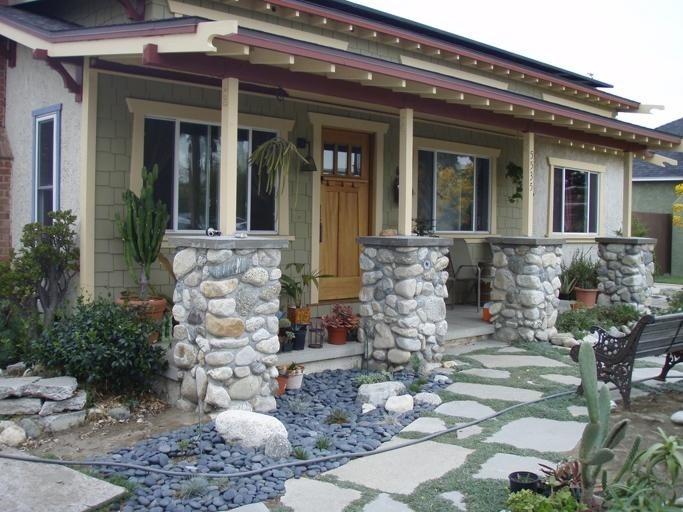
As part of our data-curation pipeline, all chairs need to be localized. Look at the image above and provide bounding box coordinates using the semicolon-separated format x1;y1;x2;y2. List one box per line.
446;237;481;312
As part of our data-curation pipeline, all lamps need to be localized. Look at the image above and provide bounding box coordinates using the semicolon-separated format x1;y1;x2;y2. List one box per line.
292;133;319;176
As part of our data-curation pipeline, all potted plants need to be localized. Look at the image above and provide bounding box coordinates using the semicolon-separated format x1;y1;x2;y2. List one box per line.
110;160;171;343
574;262;603;305
508;470;582;503
556;272;573;301
275;255;325;322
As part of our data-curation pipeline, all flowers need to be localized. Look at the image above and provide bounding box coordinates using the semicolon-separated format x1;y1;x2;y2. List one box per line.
320;304;356;329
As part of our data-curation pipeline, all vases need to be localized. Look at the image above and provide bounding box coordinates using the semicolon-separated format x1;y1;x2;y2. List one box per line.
276;375;288;395
279;326;307;352
329;324;348;346
281;365;305;390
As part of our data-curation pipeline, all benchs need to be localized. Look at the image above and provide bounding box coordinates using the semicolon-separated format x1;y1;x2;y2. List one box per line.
568;309;682;410
